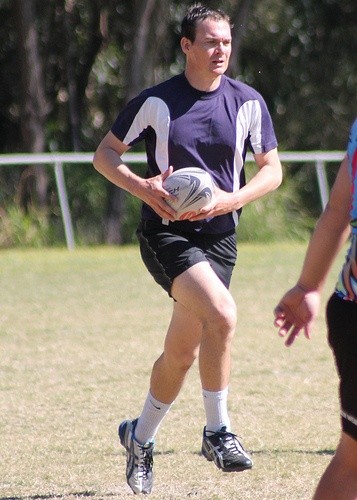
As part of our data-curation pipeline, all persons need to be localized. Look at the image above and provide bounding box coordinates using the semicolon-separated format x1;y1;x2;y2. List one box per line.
273;114;357;500
93;5;285;495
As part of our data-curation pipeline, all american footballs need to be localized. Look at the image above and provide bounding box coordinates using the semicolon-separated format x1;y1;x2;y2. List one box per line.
163;167;217;221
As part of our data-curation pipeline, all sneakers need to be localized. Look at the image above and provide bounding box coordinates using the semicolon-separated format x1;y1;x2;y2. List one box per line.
118;418;155;495
202;426;254;472
